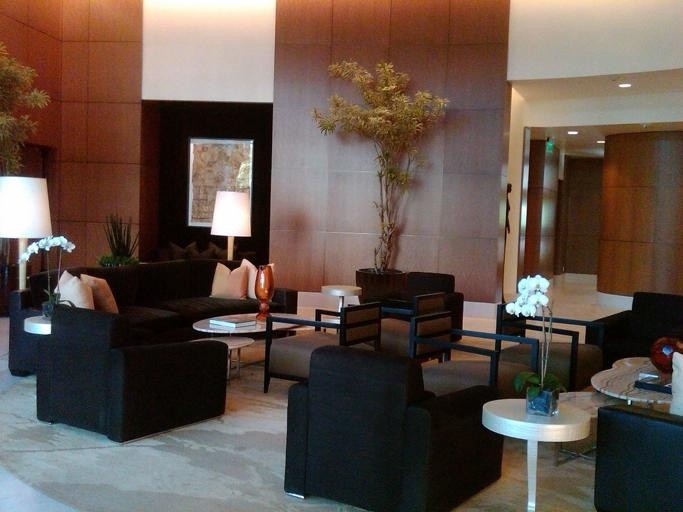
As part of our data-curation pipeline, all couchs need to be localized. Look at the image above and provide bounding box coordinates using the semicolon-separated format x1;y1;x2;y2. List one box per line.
584;284;683;511
280;342;507;511
396;273;464;343
6;259;298;381
33;305;229;444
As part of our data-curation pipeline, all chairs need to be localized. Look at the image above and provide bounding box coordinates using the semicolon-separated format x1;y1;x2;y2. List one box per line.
406;300;600;465
260;293;454;395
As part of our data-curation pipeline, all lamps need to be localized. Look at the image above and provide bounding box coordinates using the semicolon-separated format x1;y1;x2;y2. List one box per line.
0;171;59;310
207;186;252;260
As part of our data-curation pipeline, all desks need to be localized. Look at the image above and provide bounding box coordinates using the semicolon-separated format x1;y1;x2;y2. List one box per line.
192;310;308;378
482;354;676;512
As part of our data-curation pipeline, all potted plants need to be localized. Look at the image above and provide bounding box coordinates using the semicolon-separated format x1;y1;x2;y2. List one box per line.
507;273;562;418
312;58;448;300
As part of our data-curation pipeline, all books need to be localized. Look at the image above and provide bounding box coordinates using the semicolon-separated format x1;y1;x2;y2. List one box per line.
208;315;256;329
634;375;673;395
638;369;659;378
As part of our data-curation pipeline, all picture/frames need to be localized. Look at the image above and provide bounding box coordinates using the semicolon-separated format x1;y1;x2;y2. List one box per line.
185;135;254;228
